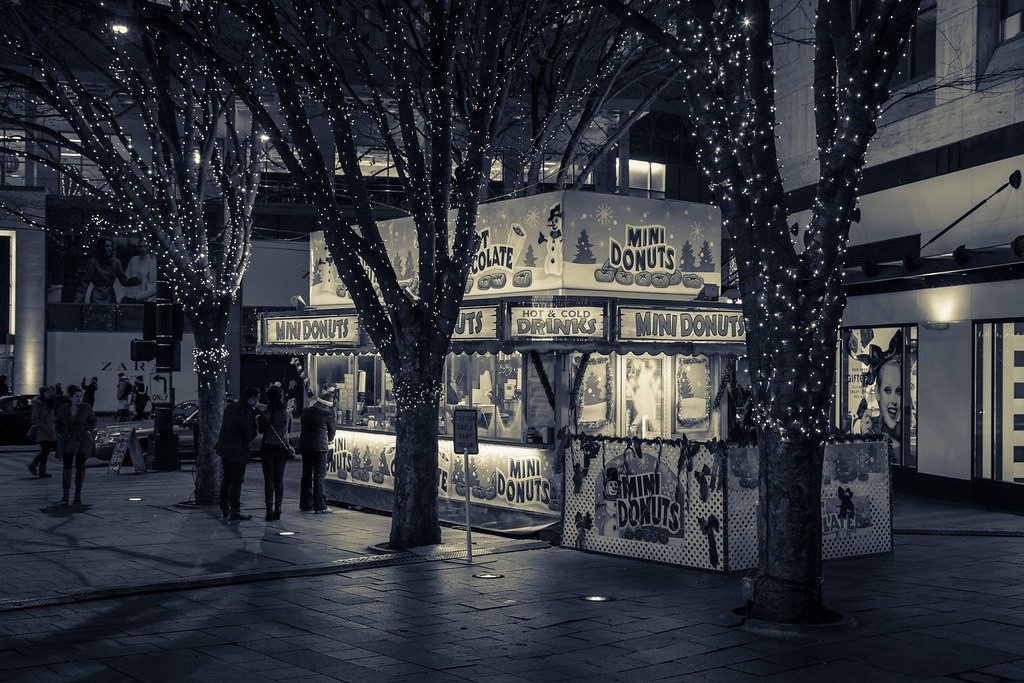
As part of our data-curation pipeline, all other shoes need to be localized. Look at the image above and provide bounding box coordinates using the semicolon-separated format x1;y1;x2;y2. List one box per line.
230;511;253;520
315;507;332;514
265;511;273;522
273;510;281;520
223;506;231;518
301;505;314;512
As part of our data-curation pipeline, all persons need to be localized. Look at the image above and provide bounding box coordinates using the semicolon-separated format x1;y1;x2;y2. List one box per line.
0;375;8;397
54;384;64;395
114;238;157;304
29;386;56;478
855;359;901;463
215;386;260;520
72;238;140;304
81;377;98;407
55;386;97;508
299;393;335;513
257;386;288;521
129;376;149;420
118;373;132;422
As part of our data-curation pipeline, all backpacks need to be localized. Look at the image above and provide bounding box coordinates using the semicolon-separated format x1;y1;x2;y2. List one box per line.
122;379;132;394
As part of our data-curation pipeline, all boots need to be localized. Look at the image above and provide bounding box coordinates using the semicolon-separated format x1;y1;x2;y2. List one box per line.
38;465;52;478
60;471;71;504
73;470;84;507
27;458;40;476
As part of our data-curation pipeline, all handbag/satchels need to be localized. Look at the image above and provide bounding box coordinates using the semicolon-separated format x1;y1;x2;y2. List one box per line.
286;446;295;459
27;424;38;441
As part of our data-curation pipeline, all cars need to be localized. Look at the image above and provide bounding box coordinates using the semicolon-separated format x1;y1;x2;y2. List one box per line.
88;399;302;470
0;392;98;443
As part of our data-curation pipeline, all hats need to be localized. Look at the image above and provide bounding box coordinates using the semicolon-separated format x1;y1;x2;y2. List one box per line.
136;376;143;382
317;393;333;406
40;386;50;395
92;377;98;384
